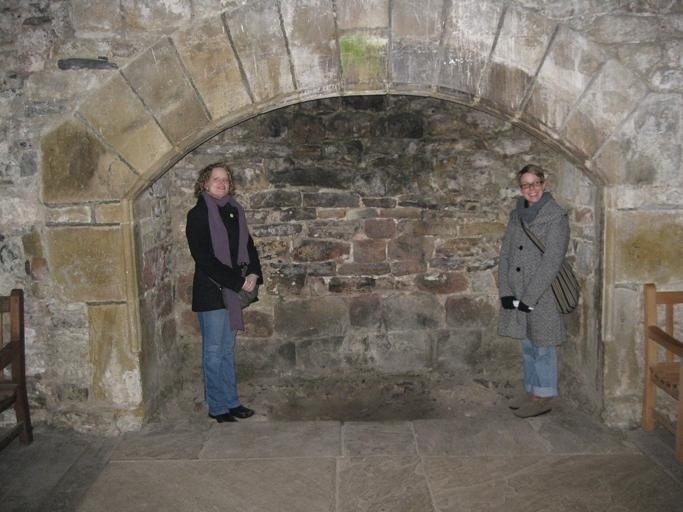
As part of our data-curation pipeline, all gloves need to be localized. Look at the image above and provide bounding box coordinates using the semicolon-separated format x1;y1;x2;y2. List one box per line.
517;300;532;313
501;296;517;309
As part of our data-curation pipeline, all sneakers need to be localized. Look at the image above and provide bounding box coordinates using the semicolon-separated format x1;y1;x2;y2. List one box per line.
208;405;254;423
508;393;552;418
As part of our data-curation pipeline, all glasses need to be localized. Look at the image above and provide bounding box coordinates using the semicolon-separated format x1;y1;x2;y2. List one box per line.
520;181;543;188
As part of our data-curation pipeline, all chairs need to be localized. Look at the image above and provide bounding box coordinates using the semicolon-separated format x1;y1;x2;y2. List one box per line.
0;288;33;444
643;283;682;459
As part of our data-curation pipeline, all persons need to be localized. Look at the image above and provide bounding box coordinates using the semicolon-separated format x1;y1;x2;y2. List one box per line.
498;164;571;417
186;162;263;422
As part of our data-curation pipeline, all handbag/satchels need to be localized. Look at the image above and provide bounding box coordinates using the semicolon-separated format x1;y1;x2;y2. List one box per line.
551;262;580;314
222;266;258;311
